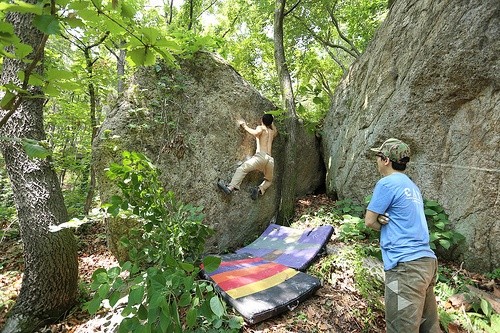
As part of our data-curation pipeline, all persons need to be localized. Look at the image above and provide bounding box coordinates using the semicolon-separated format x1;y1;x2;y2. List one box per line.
365;138;444;333
217;113;278;201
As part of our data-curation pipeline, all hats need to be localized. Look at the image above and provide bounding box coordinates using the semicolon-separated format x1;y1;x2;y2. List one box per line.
370;138;412;164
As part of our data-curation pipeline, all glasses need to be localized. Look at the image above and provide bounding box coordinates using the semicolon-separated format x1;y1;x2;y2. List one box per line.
375;154;385;161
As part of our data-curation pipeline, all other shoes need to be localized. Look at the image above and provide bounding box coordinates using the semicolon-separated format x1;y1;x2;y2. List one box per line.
251;187;258;201
217;183;232;195
